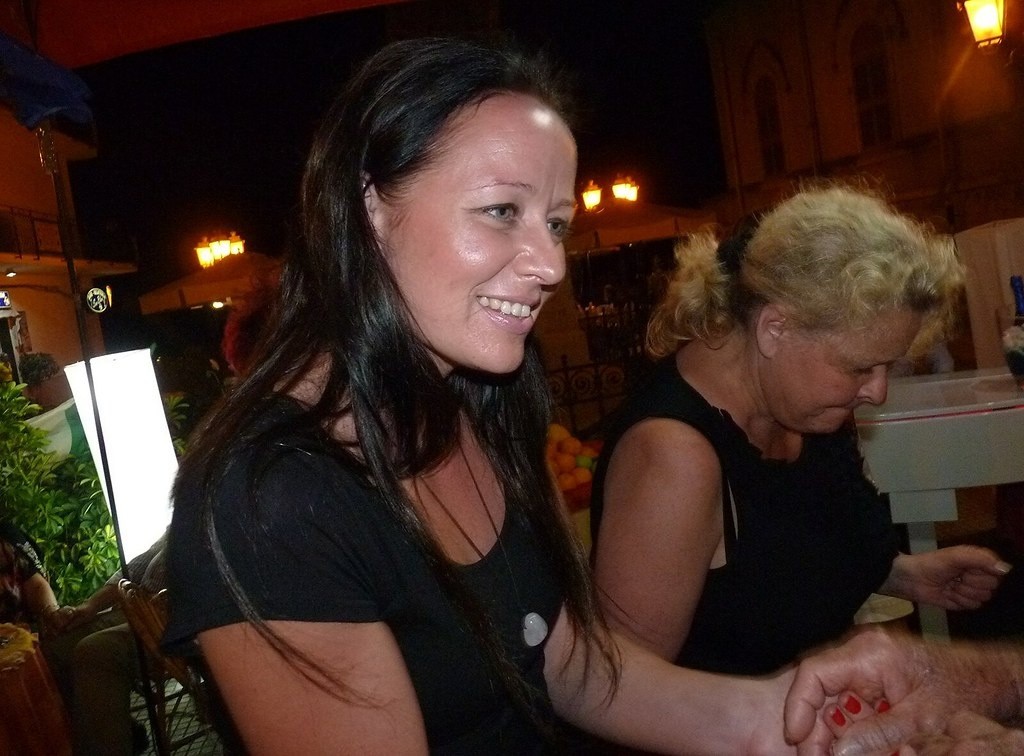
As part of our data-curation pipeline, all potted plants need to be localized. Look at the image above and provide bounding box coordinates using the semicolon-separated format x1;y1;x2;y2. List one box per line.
19;352;66;410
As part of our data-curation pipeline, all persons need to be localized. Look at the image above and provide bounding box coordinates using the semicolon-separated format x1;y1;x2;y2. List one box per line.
784;627;1024;756
164;38;590;756
590;187;1014;675
42;533;167;756
0;522;75;631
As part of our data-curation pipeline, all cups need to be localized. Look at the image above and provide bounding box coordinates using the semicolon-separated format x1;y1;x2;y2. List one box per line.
996;299;1024;391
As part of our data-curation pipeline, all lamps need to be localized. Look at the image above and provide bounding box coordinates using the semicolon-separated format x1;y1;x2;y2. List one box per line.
581;173;640;214
961;0;1007;49
193;228;250;275
63;345;179;564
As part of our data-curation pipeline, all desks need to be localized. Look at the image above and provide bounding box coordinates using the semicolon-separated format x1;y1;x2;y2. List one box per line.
0;621;76;756
853;366;1024;644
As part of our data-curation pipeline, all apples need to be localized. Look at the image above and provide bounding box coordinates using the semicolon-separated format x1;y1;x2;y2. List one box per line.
545;423;600;492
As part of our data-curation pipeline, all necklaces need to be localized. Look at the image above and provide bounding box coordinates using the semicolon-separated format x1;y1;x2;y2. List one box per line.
422;445;547;646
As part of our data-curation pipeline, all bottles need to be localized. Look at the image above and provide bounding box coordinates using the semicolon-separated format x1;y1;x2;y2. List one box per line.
1010;276;1024;326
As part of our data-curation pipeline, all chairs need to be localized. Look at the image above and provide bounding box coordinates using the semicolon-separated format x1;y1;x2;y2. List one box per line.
134;588;215;755
116;578;196;694
